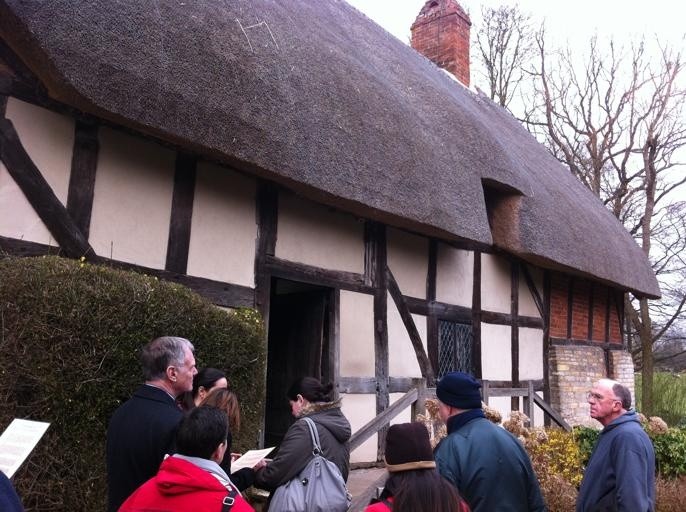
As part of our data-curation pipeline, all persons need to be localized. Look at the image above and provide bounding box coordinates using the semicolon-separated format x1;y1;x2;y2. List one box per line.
575;379;655;511
106;336;257;512
254;377;351;512
432;372;549;512
362;422;471;512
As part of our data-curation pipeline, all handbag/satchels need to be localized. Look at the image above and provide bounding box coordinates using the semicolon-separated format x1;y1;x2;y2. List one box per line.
266;415;353;512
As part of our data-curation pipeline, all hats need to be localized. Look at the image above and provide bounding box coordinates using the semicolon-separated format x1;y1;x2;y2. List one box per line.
382;421;438;472
435;370;484;410
